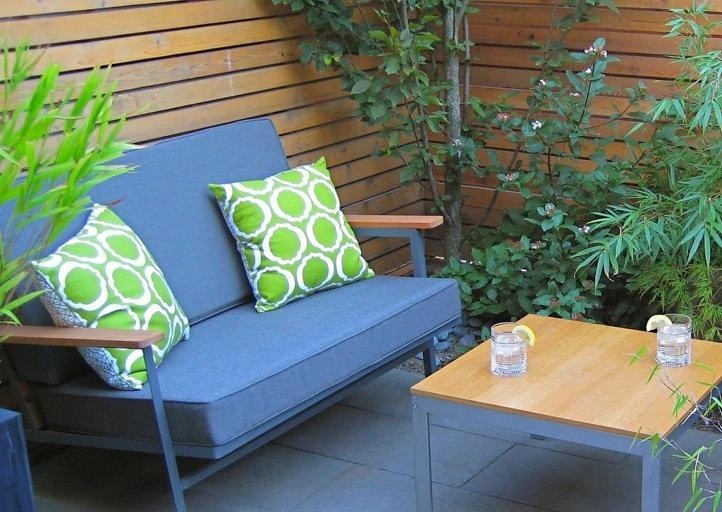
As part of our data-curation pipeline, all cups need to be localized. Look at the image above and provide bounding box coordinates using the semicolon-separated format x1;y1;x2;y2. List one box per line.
490;322;527;377
657;313;692;368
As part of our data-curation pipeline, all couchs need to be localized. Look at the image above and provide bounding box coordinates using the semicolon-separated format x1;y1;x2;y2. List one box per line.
0;118;463;512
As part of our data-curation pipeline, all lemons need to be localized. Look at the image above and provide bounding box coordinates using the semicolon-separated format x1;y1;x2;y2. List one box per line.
646;314;672;332
512;324;535;348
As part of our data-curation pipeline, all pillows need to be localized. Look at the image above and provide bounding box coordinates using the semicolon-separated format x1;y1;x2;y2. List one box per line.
27;203;191;390
208;156;375;314
